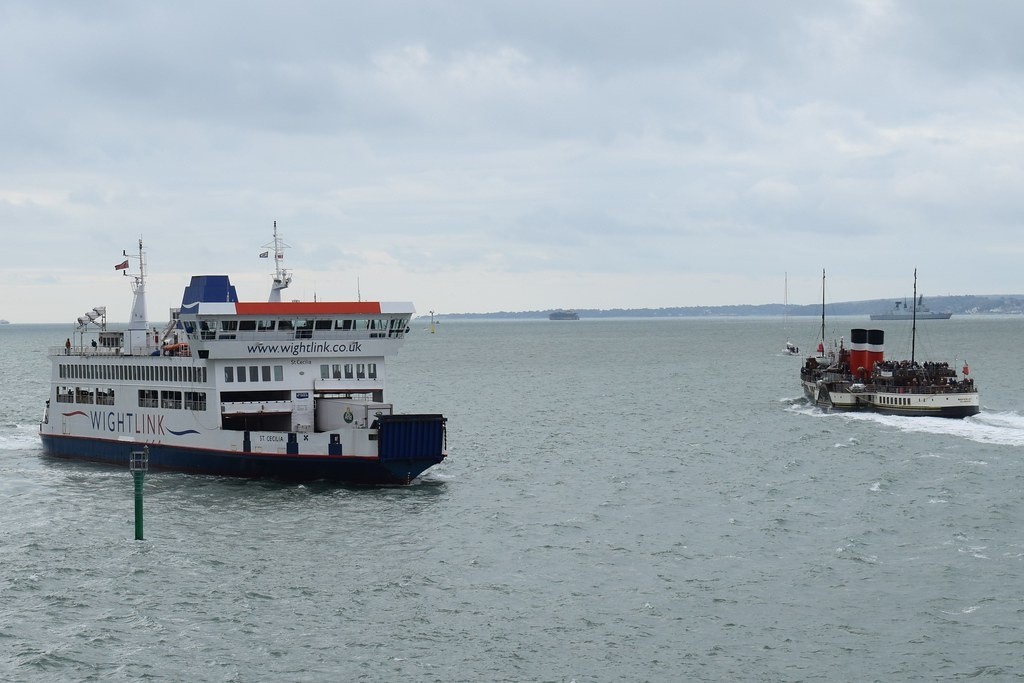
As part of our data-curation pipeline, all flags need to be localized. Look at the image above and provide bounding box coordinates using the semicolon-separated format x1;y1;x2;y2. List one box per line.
115;260;129;271
260;251;268;259
962;363;969;375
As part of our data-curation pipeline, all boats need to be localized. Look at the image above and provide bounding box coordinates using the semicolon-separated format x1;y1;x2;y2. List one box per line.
799;268;871;413
435;321;440;327
423;328;429;331
37;234;448;489
430;311;436;334
845;267;982;419
170;221;416;365
870;294;952;320
549;311;580;320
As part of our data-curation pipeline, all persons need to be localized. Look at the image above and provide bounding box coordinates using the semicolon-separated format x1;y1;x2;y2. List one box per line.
872;357;949;370
801;361;828;381
871;374;975;394
65;338;71;356
67;389;73;398
92;339;97;348
792;346;799;353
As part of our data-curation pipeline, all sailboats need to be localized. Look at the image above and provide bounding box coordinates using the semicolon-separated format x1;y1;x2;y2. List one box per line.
781;272;803;357
